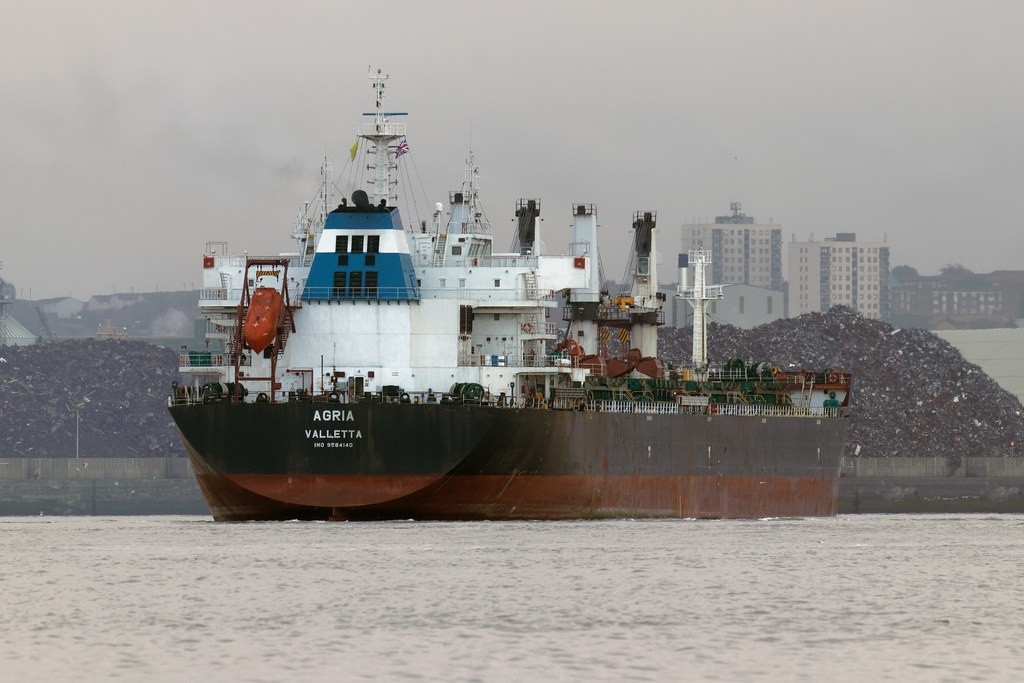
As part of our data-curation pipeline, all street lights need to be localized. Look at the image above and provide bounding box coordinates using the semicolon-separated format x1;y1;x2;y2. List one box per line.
76;403;84;460
1011;442;1014;478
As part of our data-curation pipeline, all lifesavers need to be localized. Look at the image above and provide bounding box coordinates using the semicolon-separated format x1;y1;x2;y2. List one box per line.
523;323;531;332
828;374;837;383
771;367;785;379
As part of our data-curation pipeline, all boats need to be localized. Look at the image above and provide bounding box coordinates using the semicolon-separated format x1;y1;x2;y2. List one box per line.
243;287;285;355
167;66;853;523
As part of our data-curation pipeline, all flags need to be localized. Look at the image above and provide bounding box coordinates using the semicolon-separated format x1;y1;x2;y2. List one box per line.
396;139;410;159
349;140;359;160
